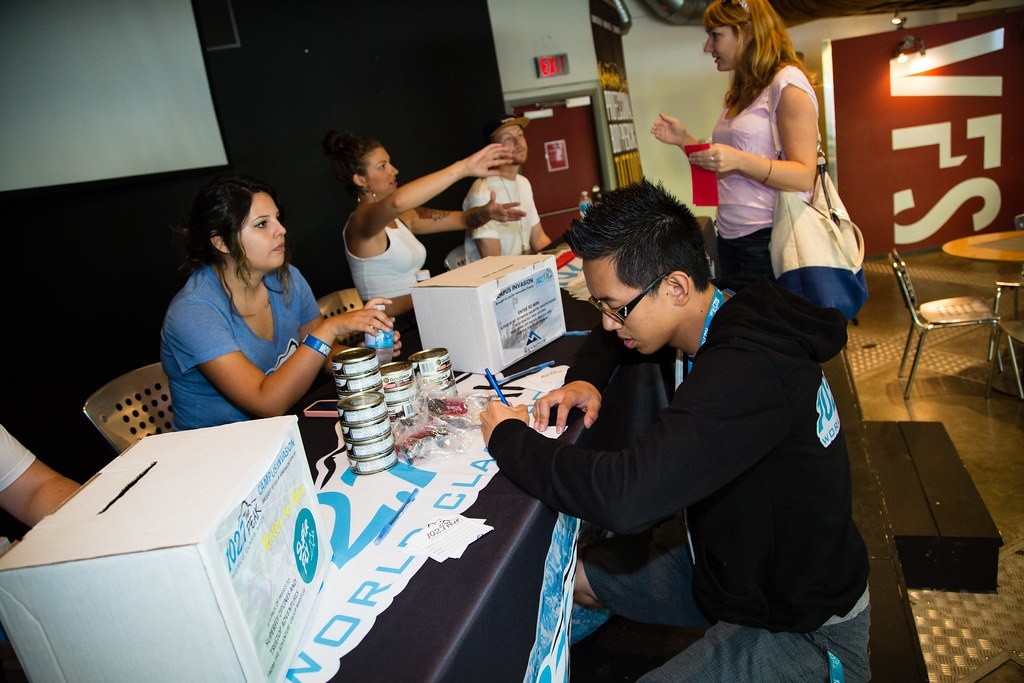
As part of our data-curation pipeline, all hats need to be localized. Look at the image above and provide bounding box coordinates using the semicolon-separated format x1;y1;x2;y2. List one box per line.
482;113;530;144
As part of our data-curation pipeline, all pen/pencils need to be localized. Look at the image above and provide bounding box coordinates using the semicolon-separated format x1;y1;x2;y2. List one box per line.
374;487;419;545
485;368;509;407
563;331;589;336
497;360;556;386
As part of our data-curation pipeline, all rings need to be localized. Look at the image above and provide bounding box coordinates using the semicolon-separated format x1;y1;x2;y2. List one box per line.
710;155;714;162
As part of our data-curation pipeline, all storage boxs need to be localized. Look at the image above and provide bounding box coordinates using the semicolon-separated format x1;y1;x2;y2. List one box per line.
409;253;567;375
0;414;333;683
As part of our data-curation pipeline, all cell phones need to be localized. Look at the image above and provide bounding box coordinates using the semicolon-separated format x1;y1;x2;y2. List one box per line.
303;400;340;418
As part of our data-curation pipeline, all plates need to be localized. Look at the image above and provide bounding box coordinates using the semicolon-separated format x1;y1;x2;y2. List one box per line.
364;304;394;367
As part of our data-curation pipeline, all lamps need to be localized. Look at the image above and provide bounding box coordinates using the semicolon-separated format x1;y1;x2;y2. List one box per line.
891;5;902;24
892;36;927;64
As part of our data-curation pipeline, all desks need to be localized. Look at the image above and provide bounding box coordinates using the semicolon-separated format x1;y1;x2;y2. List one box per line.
283;216;717;683
942;231;1024;361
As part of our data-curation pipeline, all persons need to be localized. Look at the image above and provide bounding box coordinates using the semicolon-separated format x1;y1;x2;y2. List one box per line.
0;424;82;528
478;177;874;683
158;175;402;432
650;0;820;310
462;113;552;264
321;128;526;306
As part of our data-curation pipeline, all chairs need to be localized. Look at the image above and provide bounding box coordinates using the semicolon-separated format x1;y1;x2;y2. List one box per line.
83;363;175;456
986;280;1024;401
888;247;1004;399
444;244;466;270
316;287;365;346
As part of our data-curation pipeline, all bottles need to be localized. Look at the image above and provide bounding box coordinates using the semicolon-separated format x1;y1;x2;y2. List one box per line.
416;269;431;283
591;185;602;205
580;191;593;223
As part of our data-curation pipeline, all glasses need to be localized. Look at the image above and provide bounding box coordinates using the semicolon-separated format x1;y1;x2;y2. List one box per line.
721;0;742;9
586;272;669;324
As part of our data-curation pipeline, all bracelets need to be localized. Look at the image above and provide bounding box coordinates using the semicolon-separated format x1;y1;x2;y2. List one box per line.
301;333;332;357
761;158;772;183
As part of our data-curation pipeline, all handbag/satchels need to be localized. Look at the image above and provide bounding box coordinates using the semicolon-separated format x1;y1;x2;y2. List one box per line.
769;67;868;320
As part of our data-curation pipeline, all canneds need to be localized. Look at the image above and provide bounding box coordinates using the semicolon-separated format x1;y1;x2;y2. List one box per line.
329;346;459;475
